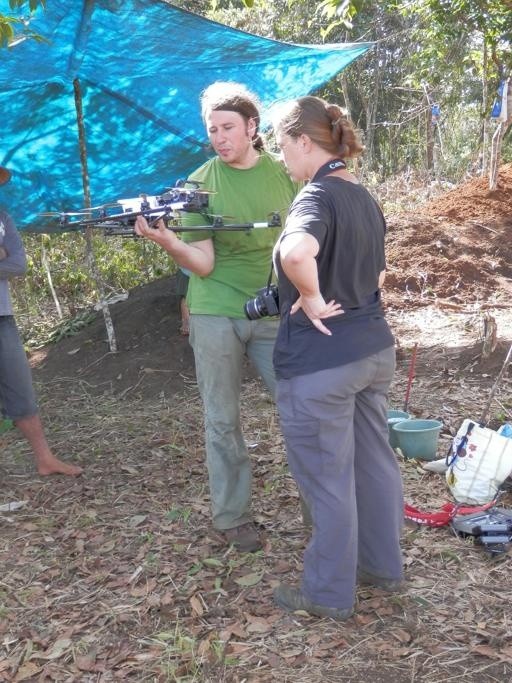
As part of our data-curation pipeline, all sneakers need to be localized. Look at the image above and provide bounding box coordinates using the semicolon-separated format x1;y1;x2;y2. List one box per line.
224;521;266;552
274;578;356;621
355;567;403;591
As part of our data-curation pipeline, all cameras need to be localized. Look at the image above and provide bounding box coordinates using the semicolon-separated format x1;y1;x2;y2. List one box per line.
244;284;279;323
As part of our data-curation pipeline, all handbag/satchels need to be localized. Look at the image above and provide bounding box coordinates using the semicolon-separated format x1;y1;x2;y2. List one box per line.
444;418;511;508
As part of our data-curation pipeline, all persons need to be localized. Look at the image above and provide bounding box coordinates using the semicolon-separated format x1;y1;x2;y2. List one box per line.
266;96;411;619
129;81;303;551
0;198;85;480
176;264;193;337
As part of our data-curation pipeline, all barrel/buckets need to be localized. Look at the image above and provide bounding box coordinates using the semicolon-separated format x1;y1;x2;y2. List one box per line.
392;420;443;461
387;409;411;448
